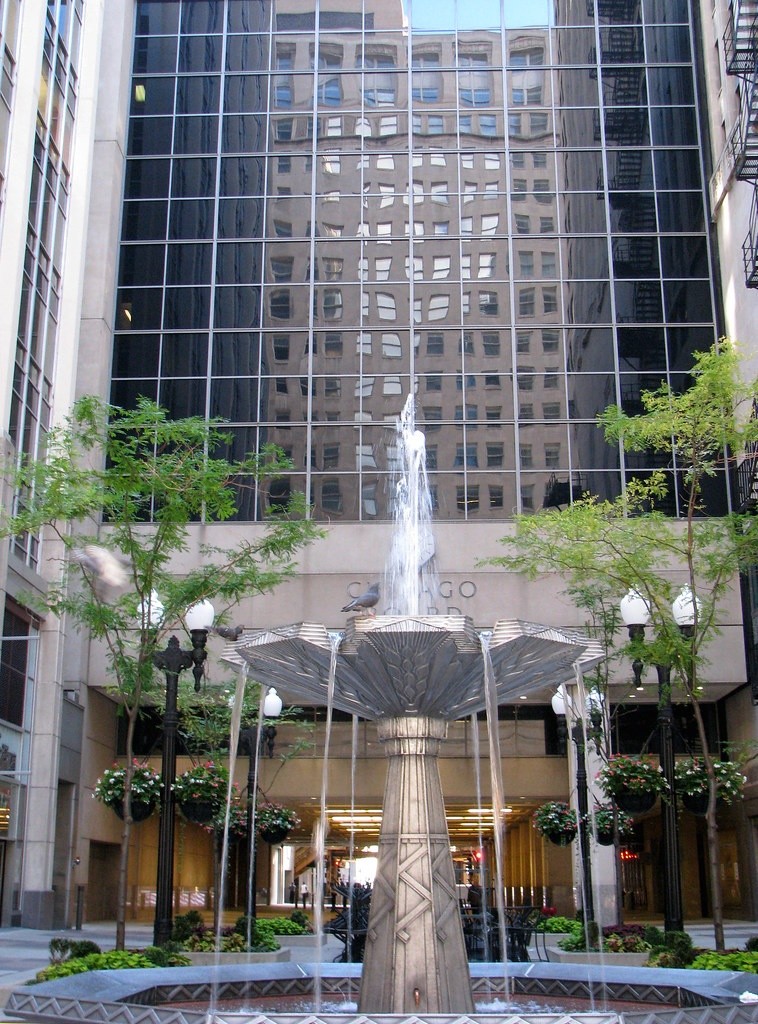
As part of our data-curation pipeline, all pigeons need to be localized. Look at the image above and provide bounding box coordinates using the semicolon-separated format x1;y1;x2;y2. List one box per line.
339;581;382;616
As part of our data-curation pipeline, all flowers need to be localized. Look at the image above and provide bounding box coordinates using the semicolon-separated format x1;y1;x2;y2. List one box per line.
169;760;248;835
254;801;300;836
530;800;583;843
582;802;634;860
558;923;664;953
593;752;671;806
532;906;582;933
674;755;748;806
91;757;164;816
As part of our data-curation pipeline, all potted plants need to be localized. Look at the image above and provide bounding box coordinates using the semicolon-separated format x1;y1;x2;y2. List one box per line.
254;909;328;946
173;910;292;967
0;938;192;1009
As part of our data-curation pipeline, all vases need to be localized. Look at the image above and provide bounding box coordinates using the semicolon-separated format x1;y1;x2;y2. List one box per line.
613;791;659;814
526;933;574;962
682;791;725;814
109;801;155;822
545;946;664;967
180;801;221;824
543;829;576;846
256;818;295;844
590;827;622;846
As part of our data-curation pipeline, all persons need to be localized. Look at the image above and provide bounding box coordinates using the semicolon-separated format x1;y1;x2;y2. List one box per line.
467;878;482;921
300;882;308;909
289;882;296;904
